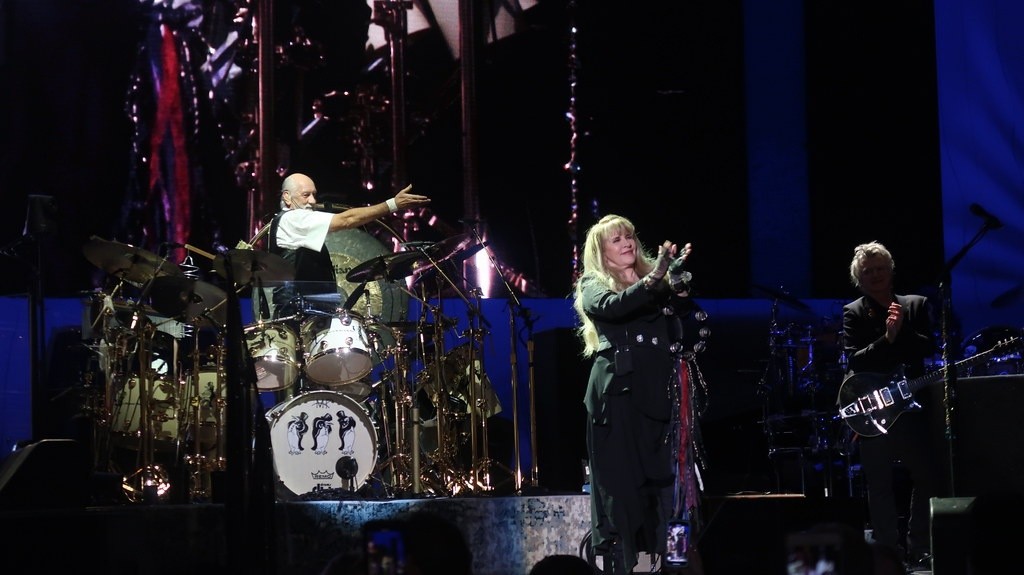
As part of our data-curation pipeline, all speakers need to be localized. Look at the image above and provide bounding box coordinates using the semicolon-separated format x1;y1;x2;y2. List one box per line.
928;497;981;575
0;438;77;505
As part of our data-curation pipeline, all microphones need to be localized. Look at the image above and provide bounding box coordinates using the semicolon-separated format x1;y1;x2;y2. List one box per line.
969;204;1003;228
163;242;185;248
406;241;436;246
457;218;484;223
213;242;231;257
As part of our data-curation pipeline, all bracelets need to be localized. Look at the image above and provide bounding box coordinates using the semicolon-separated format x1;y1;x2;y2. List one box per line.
386;198;399;212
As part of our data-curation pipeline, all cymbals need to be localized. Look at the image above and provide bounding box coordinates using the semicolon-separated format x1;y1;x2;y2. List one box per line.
369;321;432;333
423;232;473;267
141;275;229;326
213;248;297;289
83;240;185;284
344;249;429;283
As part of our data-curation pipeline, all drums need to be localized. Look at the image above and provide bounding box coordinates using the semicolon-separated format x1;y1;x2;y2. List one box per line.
304;374;374;405
109;371;187;453
244;321;299;393
180;364;229;444
268;388;379;499
416;341;504;443
300;311;374;387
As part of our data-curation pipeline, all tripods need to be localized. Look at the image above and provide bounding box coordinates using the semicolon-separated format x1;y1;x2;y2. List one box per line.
83;249;223;505
375;222;533;499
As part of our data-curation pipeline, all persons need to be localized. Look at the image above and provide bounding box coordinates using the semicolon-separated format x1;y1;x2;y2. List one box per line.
320;512;608;575
841;242;939;568
269;173;431;404
573;214;692;575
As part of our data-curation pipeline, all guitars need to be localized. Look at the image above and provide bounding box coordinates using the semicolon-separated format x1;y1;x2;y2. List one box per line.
837;335;1024;438
